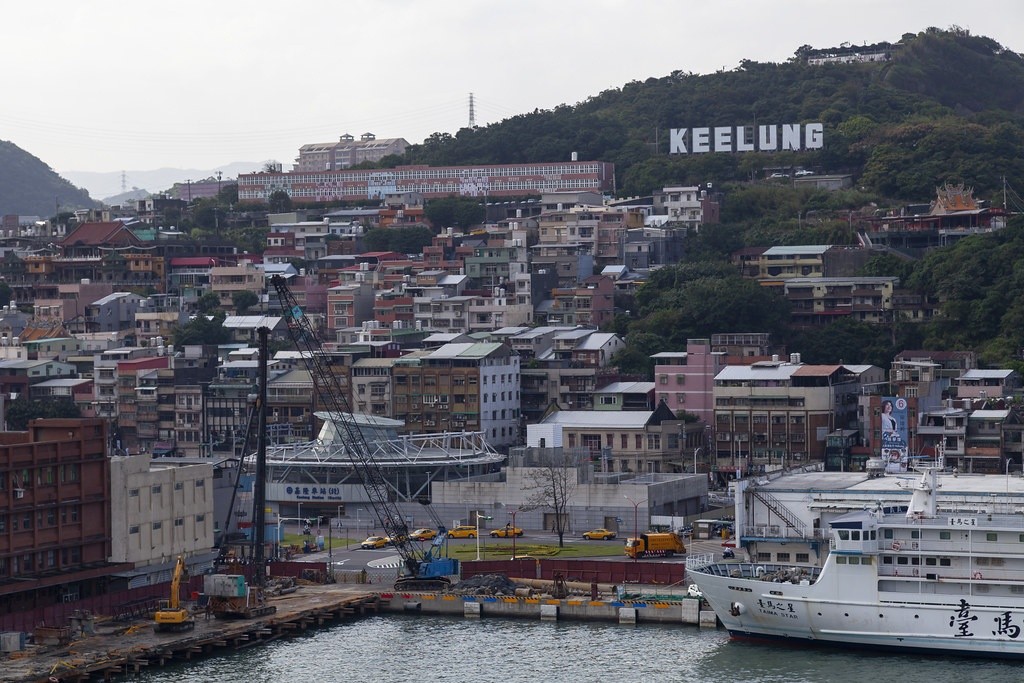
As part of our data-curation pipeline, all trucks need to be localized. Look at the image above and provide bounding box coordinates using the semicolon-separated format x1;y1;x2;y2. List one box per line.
626;533;684;560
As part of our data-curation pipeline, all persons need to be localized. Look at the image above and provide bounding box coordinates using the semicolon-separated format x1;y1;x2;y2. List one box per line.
552;520;556;533
882;401;897;434
504;526;509;538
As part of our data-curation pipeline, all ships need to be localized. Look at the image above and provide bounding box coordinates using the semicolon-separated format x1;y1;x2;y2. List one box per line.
685;434;1024;656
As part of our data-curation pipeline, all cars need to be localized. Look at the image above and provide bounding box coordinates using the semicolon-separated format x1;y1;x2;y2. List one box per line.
493;526;524;537
449;526;478;539
356;536;386;551
584;528;615;538
407;530;436;541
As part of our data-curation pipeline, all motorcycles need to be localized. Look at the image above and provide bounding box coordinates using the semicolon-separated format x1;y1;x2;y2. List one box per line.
722;551;735;557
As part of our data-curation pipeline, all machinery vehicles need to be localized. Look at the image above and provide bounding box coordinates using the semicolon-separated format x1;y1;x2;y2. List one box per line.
271;272;457;593
154;552;196;629
204;327;276;617
